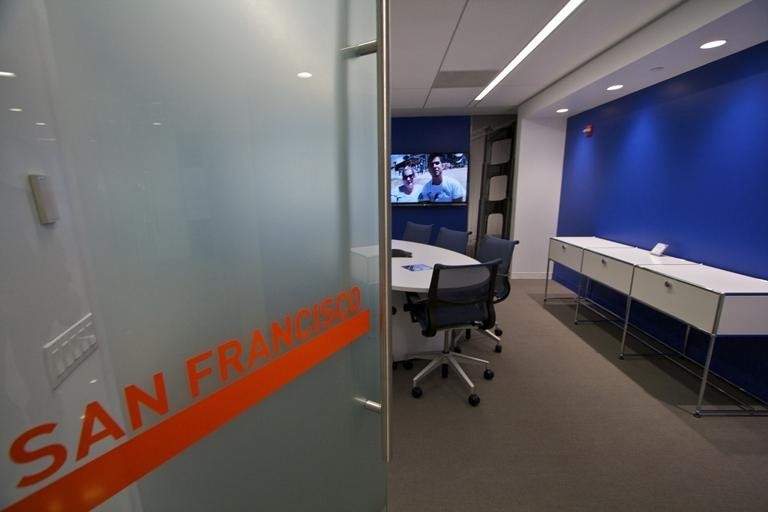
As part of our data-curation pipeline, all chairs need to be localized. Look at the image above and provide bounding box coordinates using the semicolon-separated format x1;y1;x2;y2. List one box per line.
402;220;520;406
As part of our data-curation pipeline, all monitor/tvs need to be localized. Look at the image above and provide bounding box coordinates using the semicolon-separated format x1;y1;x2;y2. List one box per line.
390;151;468;210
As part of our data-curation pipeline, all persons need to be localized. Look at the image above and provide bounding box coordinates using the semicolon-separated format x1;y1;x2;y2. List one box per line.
391;152;468;203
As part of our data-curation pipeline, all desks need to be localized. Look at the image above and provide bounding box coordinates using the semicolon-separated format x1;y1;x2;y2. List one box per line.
390;237;491;372
542;235;768;420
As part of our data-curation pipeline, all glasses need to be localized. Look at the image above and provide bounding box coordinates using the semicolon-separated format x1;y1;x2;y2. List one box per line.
403;174;414;180
